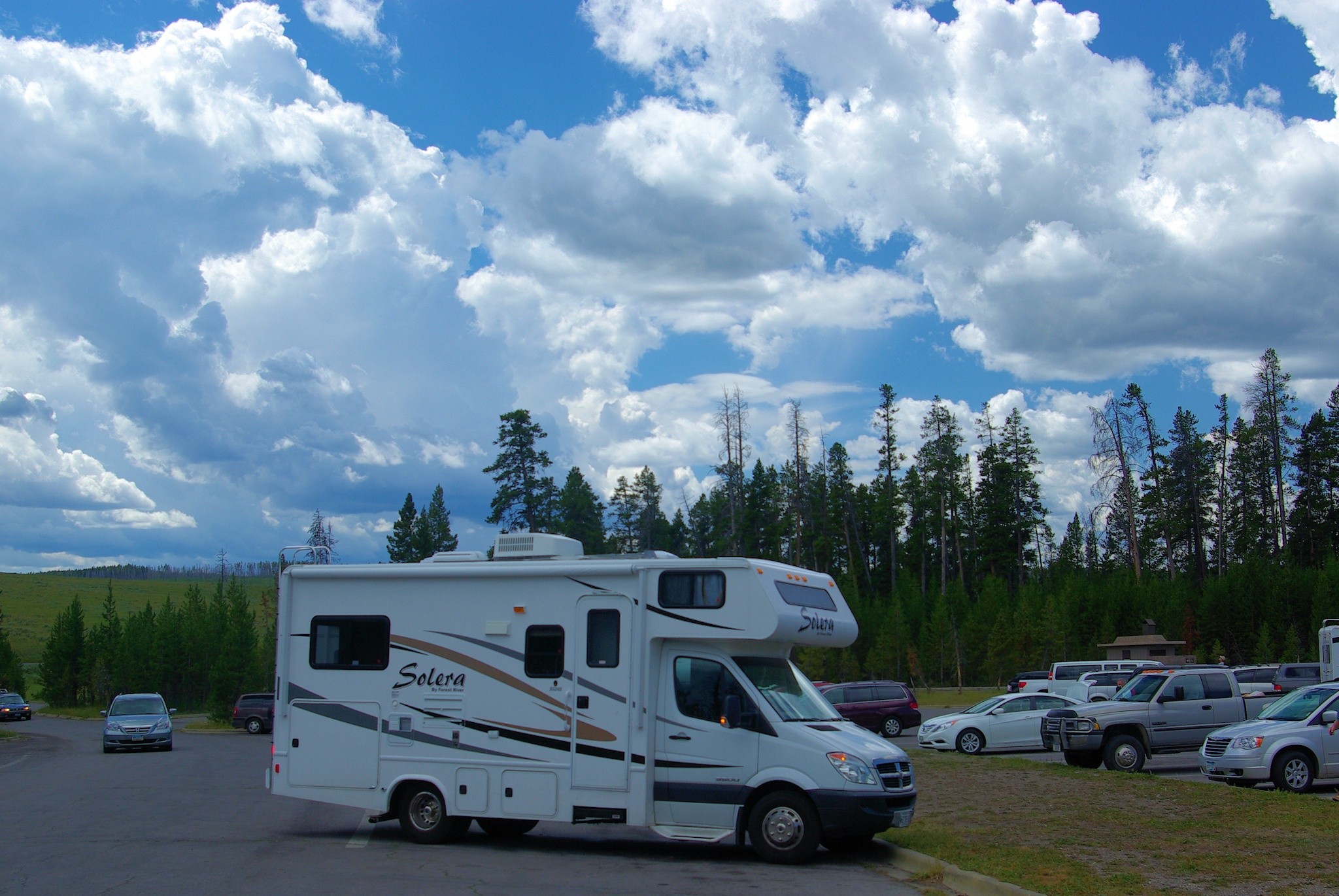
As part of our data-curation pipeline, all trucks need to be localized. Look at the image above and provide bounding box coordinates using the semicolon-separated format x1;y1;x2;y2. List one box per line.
265;532;919;864
1318;619;1339;681
1018;678;1049;694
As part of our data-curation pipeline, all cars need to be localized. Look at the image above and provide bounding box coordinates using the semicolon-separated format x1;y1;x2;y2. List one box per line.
100;692;177;753
759;682;838;696
918;680;1096;754
0;692;31;721
1198;682;1339;792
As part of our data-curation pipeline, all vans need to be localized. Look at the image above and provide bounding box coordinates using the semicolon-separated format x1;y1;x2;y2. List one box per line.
1231;664;1280;694
1048;660;1165;697
1272;663;1320;694
795;681;922;738
1077;670;1134;702
231;694;274;735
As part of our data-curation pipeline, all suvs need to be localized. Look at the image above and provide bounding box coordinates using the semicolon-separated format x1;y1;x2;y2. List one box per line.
1007;672;1049;693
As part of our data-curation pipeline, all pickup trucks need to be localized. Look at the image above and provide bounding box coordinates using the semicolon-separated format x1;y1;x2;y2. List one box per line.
1039;668;1321;773
